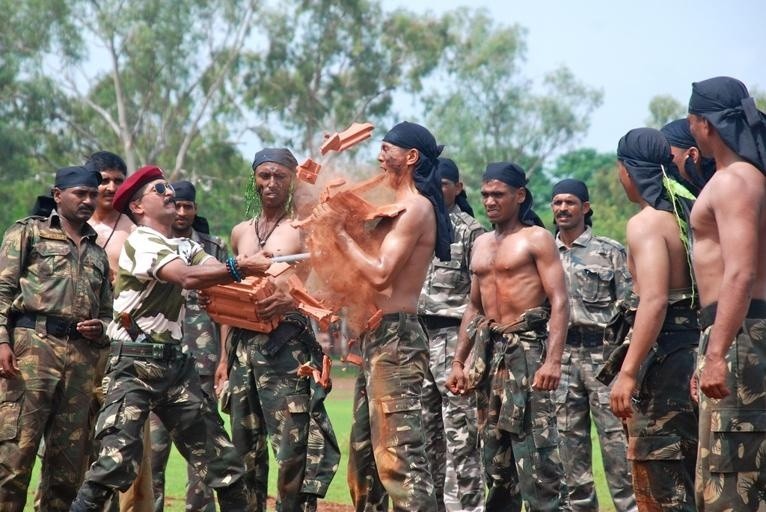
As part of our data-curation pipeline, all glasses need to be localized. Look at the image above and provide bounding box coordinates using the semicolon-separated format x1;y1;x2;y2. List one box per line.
151;182;175;194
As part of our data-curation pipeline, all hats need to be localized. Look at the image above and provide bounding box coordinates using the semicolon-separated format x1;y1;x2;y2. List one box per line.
111;166;165;212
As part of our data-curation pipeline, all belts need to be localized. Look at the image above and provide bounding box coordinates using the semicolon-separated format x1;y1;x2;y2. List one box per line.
16;312;83;339
109;340;182;362
694;299;765;332
419;315;457;330
564;326;604;348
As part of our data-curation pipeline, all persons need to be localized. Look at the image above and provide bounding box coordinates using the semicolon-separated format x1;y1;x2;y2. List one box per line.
1;148;343;510
310;75;766;512
